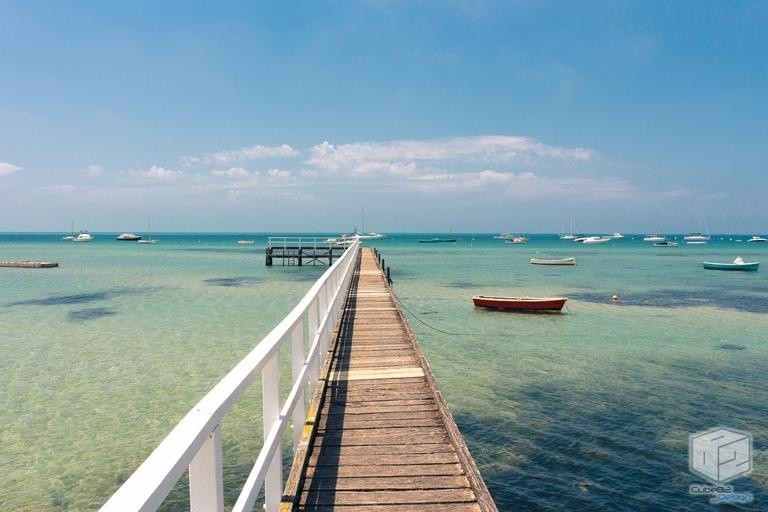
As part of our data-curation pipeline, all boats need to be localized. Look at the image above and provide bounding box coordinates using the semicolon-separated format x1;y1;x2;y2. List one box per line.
117;233;157;244
472;295;567;312
644;232;710;247
325;233;382;247
561;233;623;243
500;232;528;244
703;262;760;270
748;237;766;241
418;238;456;243
530;257;576;265
63;232;95;242
